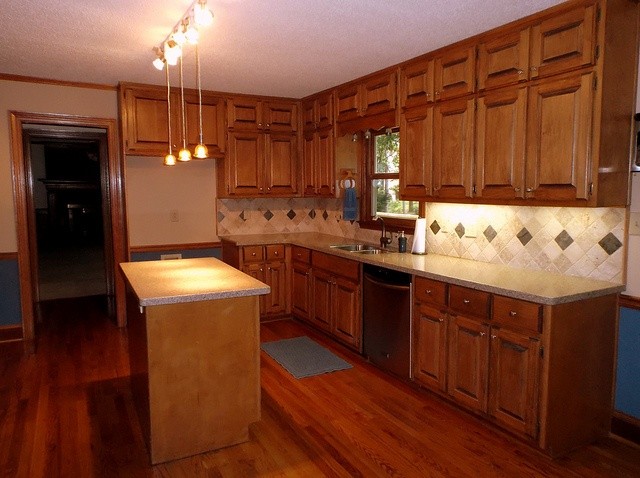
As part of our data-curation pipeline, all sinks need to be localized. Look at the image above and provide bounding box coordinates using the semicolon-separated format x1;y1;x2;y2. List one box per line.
351;250;391;255
330;245;372;251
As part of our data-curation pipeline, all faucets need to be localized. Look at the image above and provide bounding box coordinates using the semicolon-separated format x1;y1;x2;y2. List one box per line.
372;215;393;247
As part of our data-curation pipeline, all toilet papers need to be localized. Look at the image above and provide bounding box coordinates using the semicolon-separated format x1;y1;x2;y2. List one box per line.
410;218;426;254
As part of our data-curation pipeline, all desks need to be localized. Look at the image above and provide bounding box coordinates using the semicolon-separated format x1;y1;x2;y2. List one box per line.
119;257;269;466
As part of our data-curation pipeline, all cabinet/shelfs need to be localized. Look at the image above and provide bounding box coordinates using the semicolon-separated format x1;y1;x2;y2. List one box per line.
445;283;542;446
412;275;447;401
477;1;640;208
219;92;305;198
116;80;225;159
398;34;476;204
290;245;312;327
333;66;399;123
312;249;361;353
301;87;334;199
237;245;290;320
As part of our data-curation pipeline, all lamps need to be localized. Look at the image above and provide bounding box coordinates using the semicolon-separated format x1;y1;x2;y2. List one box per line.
151;0;213;166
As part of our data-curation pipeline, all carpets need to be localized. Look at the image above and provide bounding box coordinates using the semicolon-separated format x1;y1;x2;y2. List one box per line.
364;341;392;364
262;335;351;379
